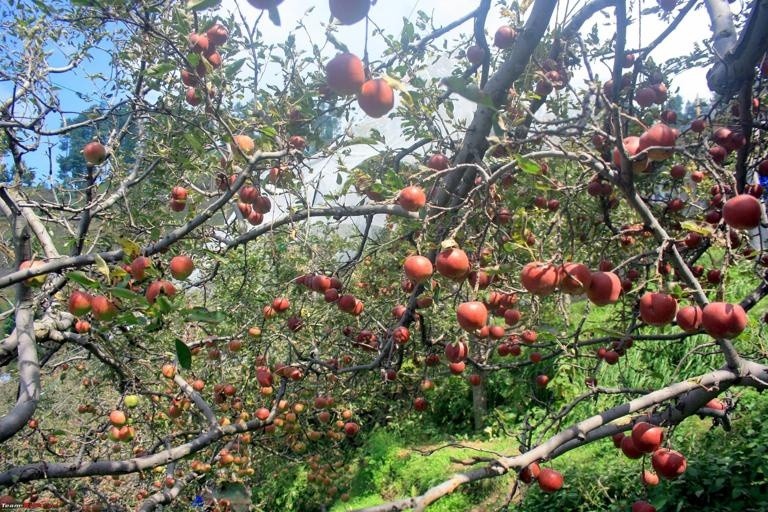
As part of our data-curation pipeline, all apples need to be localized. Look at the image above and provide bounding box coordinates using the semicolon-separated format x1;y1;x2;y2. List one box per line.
1;1;768;512
